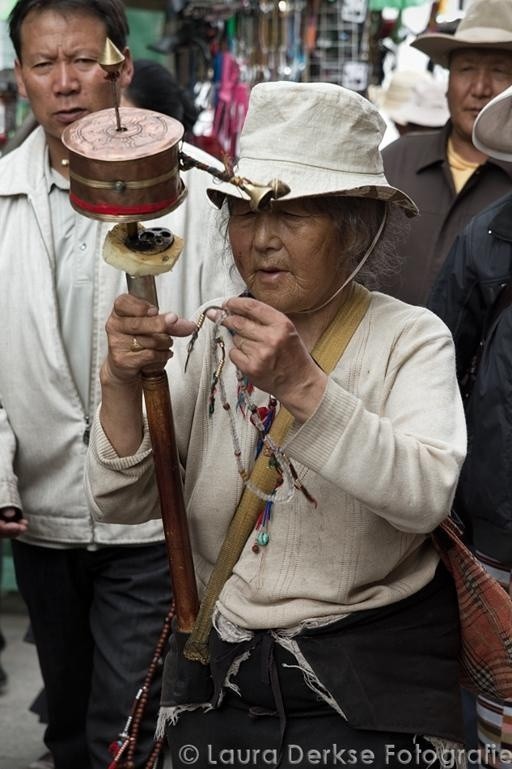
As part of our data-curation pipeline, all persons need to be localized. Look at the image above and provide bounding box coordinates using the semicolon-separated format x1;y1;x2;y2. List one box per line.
121;58;196;144
79;81;466;769
382;0;511;594
0;0;244;769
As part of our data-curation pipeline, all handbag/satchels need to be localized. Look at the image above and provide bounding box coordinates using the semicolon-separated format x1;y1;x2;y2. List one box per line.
439;517;511;699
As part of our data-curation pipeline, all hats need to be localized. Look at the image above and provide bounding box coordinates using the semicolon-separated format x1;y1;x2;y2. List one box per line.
209;1;512;214
